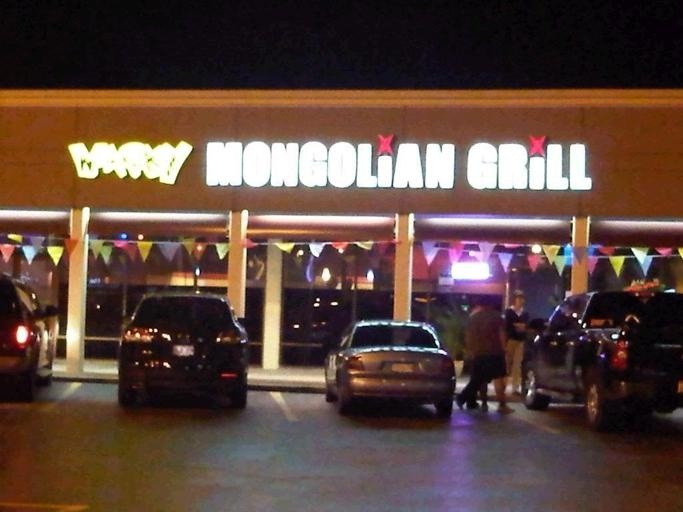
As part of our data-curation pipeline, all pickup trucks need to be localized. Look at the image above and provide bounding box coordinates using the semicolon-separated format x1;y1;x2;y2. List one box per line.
520;291;683;430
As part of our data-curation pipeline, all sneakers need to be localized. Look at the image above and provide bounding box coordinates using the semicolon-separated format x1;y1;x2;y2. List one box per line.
458;391;522;414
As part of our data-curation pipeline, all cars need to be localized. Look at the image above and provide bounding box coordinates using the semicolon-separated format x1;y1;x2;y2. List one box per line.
1;274;59;398
118;293;249;407
323;320;456;420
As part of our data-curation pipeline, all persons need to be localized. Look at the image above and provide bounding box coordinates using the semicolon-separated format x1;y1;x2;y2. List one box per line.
499;290;529;397
467;296;535;413
454;296;526;411
